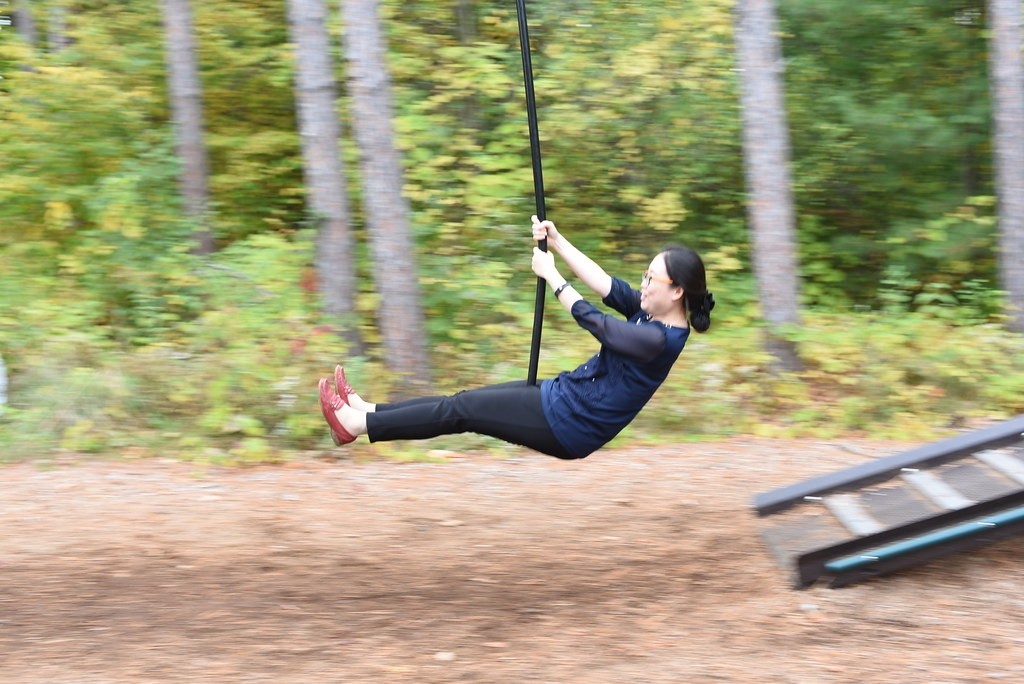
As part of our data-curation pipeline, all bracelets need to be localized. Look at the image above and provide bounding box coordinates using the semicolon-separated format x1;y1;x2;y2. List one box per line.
555;283;569;298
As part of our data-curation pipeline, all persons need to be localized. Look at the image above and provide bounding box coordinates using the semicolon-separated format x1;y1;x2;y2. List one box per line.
319;215;714;460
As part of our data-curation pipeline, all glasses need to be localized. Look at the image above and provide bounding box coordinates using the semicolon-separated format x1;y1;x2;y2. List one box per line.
642;272;673;286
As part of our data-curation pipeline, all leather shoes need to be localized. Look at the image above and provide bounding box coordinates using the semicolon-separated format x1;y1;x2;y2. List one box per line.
318;378;357;446
335;365;356;407
